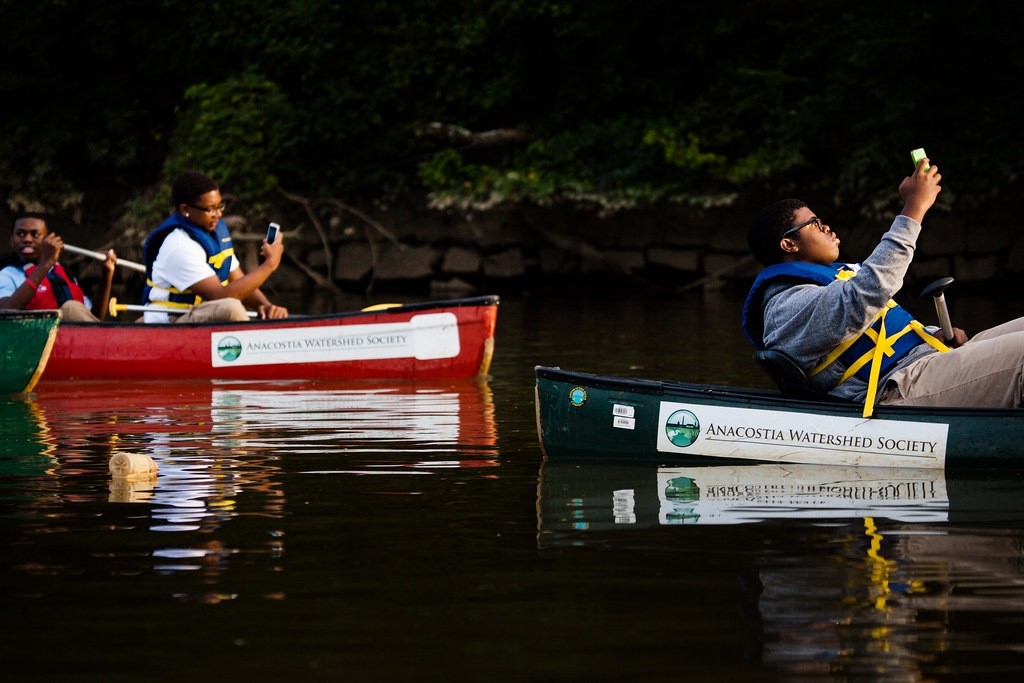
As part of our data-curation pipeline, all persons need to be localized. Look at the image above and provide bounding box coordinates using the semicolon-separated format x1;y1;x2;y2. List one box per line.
743;158;1024;407
0;212;118;324
134;172;289;323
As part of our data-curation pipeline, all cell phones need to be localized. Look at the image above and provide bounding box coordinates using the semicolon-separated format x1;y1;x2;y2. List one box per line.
911;148;931;173
265;223;280;244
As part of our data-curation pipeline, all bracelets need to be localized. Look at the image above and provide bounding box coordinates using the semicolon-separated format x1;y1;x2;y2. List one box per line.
25;279;37;291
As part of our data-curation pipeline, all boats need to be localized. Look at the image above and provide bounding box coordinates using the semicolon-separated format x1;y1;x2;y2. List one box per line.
530;364;1023;482
1;307;66;402
534;453;1023;550
38;380;496;451
1;392;61;481
37;292;504;382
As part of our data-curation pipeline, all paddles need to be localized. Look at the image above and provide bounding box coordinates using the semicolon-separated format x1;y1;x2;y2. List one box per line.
926;276;960;348
105;297;404;316
59;243;147;274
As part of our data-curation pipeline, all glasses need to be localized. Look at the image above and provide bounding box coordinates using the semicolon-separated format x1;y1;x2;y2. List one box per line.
781;218;822;240
187;201;226;212
